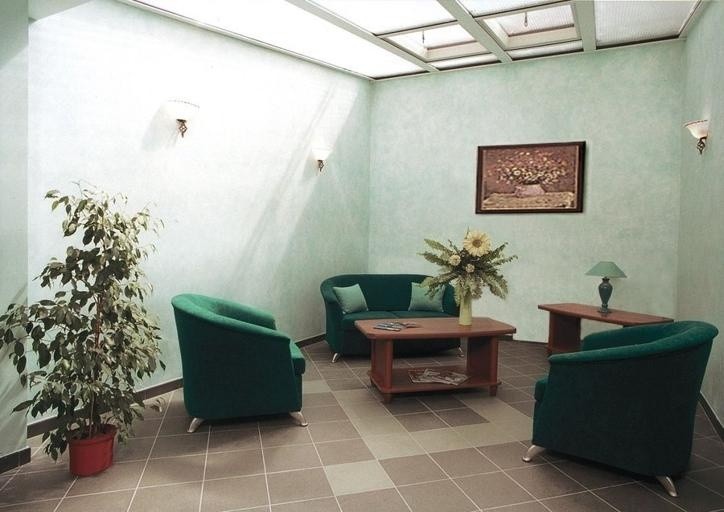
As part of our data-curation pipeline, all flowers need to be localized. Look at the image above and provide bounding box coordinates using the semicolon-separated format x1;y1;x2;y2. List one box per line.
415;223;522;305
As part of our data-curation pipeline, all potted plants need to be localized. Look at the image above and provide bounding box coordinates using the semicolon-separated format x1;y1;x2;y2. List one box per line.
0;180;167;476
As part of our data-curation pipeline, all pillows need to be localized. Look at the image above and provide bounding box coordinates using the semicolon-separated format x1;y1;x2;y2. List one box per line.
407;279;447;315
332;282;372;315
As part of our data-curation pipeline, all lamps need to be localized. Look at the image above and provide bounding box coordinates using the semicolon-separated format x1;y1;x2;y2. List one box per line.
584;260;628;315
164;97;198;138
682;118;712;156
313;144;335;173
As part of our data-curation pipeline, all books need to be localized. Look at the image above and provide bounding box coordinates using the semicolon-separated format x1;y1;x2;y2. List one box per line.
408;368;470;386
375;319;421;331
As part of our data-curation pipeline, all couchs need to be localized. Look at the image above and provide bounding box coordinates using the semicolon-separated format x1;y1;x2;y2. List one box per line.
520;319;720;497
172;294;307;434
320;271;459;364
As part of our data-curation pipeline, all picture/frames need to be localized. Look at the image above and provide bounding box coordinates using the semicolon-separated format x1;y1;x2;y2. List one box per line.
474;139;586;216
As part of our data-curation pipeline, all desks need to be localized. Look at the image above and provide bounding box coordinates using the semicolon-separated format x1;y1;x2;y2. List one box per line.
537;301;673;359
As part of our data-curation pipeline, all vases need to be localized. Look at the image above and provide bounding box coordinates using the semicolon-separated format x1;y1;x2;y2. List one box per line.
456;290;475;328
71;424;114;477
